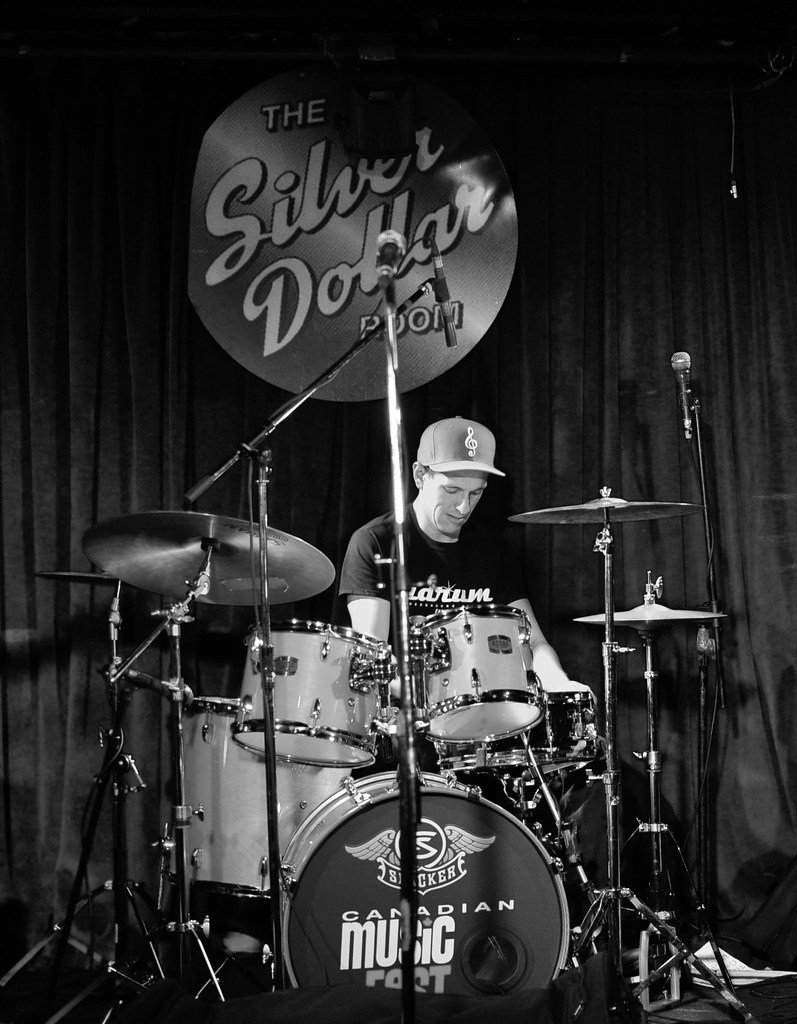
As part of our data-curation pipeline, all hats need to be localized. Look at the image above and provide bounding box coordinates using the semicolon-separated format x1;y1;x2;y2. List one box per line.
416;415;506;477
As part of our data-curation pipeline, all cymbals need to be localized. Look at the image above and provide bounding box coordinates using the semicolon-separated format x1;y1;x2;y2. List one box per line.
78;509;336;614
502;497;703;527
571;601;723;626
34;567;130;592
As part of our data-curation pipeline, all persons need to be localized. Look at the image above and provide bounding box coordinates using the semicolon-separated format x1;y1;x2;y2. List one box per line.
338;413;644;973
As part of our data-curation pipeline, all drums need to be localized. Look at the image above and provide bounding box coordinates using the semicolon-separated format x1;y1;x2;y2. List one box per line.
437;685;602;776
274;770;573;994
166;692;356;903
416;601;545;745
231;618;381;770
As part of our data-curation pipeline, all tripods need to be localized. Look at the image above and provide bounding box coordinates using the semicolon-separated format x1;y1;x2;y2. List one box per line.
566;512;760;1024
0;550;228;1024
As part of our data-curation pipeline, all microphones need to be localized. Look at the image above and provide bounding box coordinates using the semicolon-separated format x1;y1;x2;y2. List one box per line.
119;668;194;707
431;240;458;348
375;230;408;287
670;352;695;438
